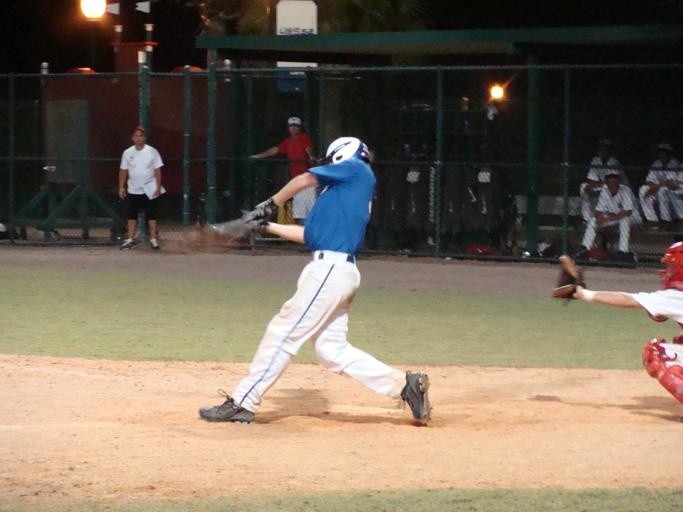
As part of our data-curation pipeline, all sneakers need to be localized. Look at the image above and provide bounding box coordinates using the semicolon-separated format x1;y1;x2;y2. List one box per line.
118;237;139;251
399;369;430;426
148;235;157;249
197;389;254;423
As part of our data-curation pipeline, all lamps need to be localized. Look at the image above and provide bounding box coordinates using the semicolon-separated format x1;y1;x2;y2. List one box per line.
80;0;106;22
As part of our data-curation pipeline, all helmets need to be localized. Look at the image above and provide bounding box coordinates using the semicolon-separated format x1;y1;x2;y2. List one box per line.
326;136;374;165
658;242;683;292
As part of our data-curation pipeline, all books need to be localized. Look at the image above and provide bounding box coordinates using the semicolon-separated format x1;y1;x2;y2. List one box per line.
140;177;166;201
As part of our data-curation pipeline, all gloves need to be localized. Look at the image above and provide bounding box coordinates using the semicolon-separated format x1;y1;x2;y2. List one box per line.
241;198;279;221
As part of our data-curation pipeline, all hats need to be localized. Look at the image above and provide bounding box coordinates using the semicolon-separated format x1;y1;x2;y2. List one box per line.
128;127;144;137
286;116;301;125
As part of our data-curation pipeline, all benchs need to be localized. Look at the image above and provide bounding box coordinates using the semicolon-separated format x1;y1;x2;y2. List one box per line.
516;194;683;258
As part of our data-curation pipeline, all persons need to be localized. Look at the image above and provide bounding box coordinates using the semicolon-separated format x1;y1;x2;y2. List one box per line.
550;239;682;416
655;160;682;221
116;127;161;252
577;138;631;232
195;136;435;426
248;115;320;256
567;169;645;263
637;142;679;231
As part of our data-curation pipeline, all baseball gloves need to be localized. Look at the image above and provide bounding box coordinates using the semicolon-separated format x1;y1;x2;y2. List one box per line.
551;255;586;300
